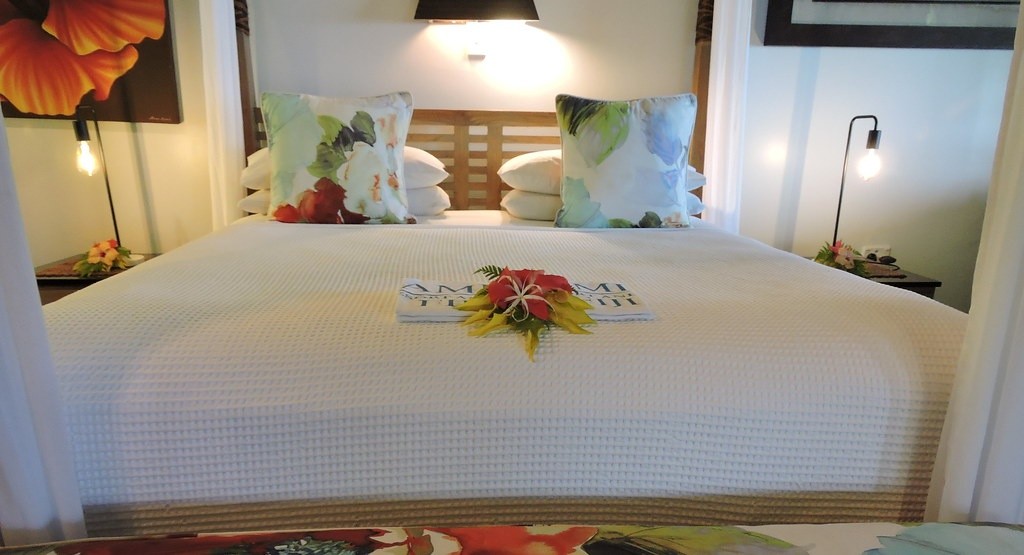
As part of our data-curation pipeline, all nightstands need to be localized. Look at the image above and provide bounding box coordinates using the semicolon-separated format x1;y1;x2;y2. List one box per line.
805;256;943;299
32;252;162;305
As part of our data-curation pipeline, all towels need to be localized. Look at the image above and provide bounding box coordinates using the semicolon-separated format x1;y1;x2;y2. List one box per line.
395;279;653;324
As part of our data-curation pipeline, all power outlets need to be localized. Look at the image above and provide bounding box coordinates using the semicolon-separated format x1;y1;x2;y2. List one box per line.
863;245;892;260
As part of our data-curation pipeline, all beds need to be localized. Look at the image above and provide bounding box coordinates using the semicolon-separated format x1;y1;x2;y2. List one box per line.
37;108;970;532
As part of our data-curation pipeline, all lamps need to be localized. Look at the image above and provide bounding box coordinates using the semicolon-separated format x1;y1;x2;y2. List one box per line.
834;114;884;244
73;104;146;268
414;0;541;22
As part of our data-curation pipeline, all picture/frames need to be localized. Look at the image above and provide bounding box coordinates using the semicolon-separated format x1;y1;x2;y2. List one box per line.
764;0;1020;51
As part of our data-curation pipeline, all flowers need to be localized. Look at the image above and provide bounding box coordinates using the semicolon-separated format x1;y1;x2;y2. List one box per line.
814;241;872;279
73;237;133;277
458;265;596;360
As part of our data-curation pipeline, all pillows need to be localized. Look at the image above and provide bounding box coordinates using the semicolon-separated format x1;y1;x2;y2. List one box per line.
554;93;698;230
496;149;708;196
237;184;451;216
240;143;450;191
500;189;707;221
260;91;421;224
